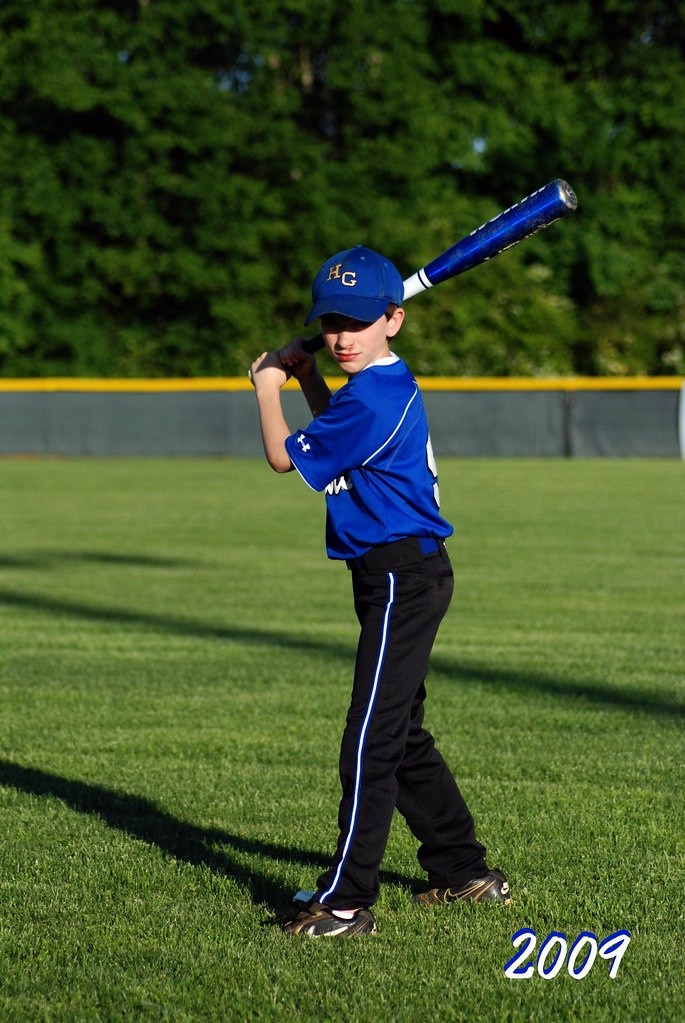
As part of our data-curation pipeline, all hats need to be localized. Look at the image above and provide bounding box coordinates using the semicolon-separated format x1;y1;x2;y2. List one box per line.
303;243;405;325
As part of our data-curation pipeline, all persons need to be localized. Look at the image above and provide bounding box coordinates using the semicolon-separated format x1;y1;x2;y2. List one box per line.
247;247;508;939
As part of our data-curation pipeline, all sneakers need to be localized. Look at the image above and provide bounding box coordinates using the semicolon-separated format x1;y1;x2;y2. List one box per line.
409;870;514;909
275;891;378;938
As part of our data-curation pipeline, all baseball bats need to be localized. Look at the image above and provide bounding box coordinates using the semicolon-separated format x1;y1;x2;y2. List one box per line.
246;178;578;389
